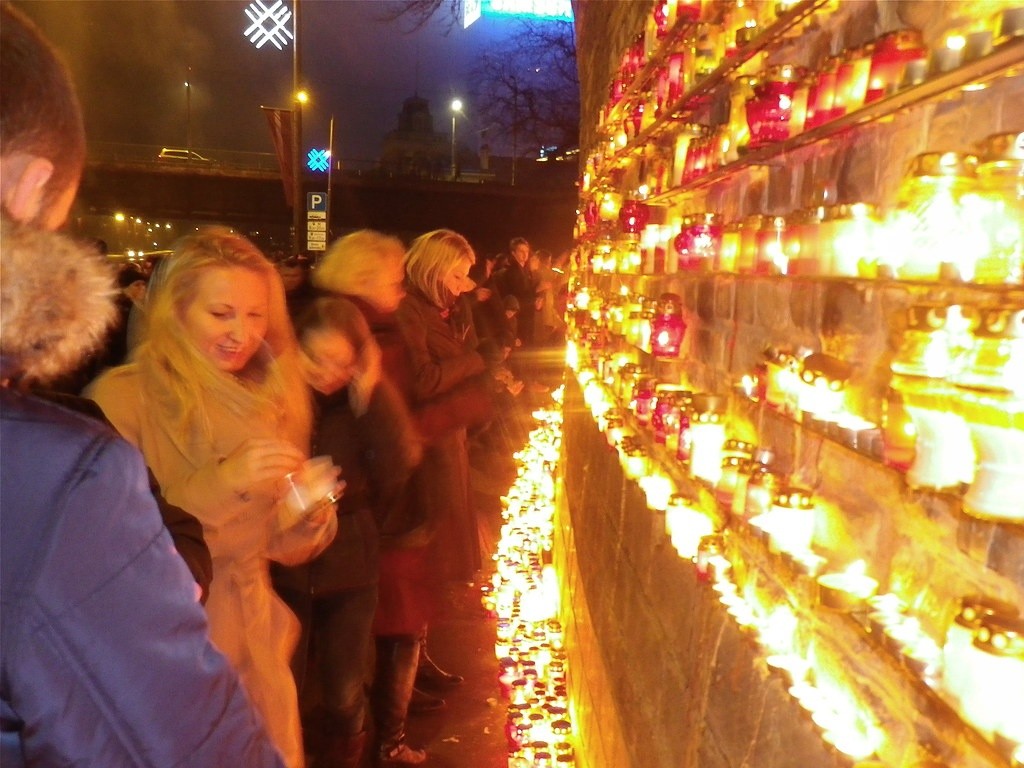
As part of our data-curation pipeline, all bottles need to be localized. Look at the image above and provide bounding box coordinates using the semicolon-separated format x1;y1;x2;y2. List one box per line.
480;0;1024;768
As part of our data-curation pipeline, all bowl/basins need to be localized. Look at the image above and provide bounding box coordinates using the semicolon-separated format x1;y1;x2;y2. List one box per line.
275;456;345;520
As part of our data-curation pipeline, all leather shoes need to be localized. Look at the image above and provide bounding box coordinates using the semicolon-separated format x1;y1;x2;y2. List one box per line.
409;687;445;713
418;653;464;685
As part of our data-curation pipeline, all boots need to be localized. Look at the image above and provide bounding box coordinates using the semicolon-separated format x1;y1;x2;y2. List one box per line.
321;730;364;768
371;641;425;766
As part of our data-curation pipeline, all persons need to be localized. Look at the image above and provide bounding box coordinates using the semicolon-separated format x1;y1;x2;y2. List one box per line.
0;0;283;768
269;229;571;768
88;237;159;363
81;224;337;768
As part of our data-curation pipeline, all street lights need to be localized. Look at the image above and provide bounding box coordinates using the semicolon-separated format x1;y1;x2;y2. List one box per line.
452;101;460;178
184;64;191;167
